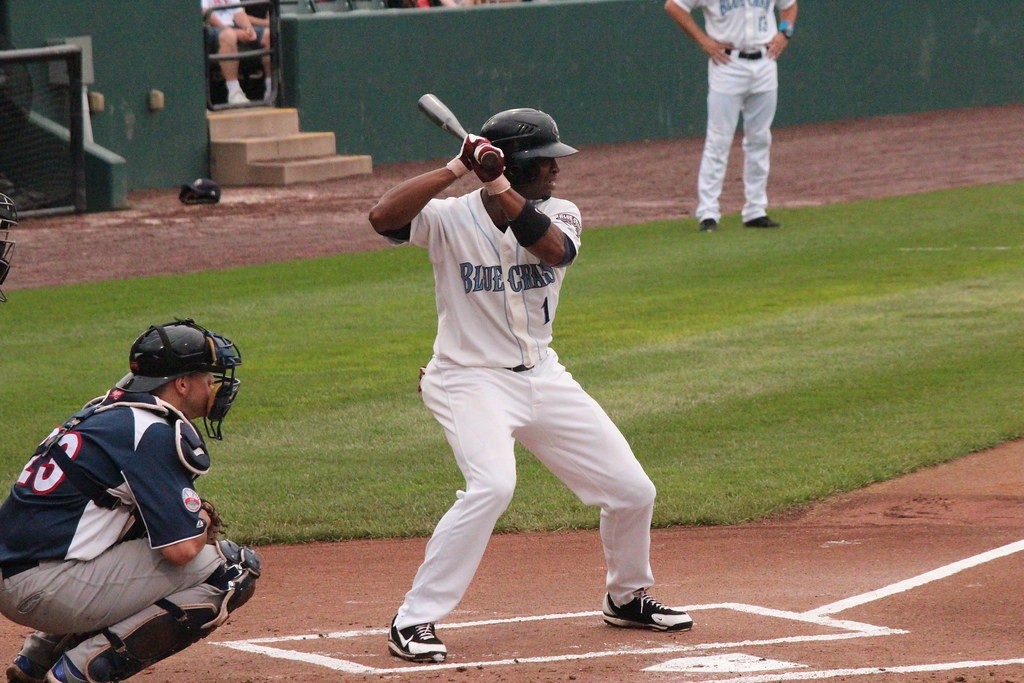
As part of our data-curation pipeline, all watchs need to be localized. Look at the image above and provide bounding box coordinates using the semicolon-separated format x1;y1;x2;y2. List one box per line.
781;30;793;38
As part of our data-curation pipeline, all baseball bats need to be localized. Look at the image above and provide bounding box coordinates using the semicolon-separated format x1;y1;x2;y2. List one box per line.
416;92;500;170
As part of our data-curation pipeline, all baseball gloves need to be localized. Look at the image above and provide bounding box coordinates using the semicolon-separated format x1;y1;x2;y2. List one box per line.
199;498;230;547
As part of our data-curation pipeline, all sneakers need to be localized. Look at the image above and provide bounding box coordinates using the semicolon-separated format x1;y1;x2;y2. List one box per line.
602;588;693;632
388;614;447;661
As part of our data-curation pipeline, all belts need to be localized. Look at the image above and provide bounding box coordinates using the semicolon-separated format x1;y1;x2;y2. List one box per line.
724;48;762;60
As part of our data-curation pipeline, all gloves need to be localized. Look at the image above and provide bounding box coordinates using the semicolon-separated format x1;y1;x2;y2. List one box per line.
465;141;511;196
446;133;490;180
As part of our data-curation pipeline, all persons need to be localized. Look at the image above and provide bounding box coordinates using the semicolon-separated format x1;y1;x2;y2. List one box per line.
664;0;799;233
0;317;261;683
369;108;693;664
201;0;272;104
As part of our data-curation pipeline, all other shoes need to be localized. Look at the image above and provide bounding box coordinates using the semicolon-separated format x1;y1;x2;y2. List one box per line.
263;91;272;101
228;90;249;104
44;653;90;683
699;219;717;232
6;655;49;683
745;216;778;228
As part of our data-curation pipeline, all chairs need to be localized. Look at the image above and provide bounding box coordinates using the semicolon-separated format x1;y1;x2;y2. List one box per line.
272;0;391;16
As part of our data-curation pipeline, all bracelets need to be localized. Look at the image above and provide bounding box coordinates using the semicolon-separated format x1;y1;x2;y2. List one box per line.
446;157;470;179
483;173;511;196
778;21;794;32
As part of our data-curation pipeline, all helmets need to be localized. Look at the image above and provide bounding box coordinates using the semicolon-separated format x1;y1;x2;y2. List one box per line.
116;321;217;393
0;192;14;283
480;107;579;161
